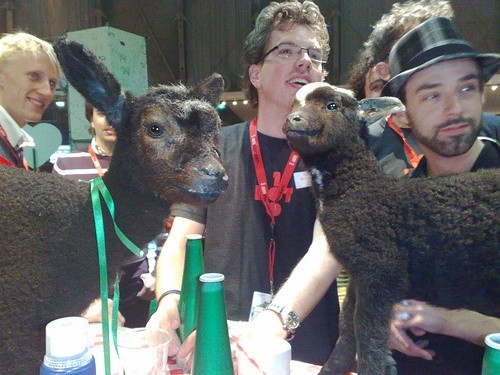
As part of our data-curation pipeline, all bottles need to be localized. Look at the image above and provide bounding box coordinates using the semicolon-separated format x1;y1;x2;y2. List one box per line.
481;332;500;375
176;233;207;345
191;272;234;375
37;316;97;374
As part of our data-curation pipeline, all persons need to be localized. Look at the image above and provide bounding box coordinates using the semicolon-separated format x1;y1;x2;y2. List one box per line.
0;31;63;170
349;0;500;375
51;99;171;328
146;0;346;370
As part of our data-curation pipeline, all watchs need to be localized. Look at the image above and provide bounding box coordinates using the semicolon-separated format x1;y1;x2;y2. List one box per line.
265;301;302;340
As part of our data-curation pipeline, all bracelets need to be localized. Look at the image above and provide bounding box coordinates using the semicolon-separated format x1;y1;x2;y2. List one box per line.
157;290;182;308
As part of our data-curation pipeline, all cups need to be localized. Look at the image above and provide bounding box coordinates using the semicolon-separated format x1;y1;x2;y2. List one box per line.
236;336;292;374
113;326;171;375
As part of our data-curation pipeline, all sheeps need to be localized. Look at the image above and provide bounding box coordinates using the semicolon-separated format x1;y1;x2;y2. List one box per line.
282;81;500;375
0;40;229;375
